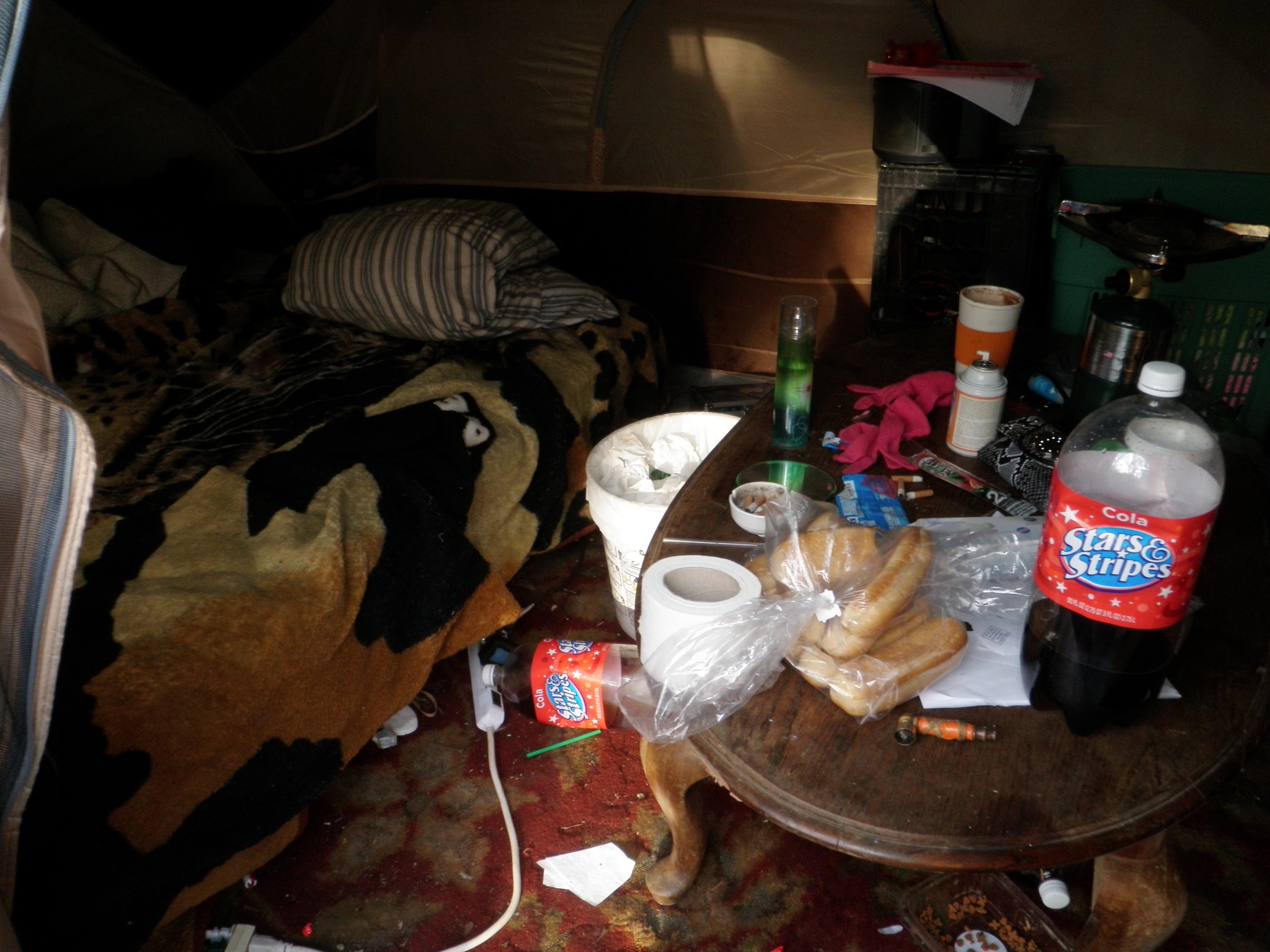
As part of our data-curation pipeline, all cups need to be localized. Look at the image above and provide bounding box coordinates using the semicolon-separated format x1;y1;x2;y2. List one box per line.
774;291;818;450
950;285;1025;385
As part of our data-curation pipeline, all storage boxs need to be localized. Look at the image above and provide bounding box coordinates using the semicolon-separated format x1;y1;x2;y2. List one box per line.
1049;205;1268;445
870;143;1067;339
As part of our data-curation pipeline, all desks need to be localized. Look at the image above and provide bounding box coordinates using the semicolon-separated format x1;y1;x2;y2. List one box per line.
630;331;1270;950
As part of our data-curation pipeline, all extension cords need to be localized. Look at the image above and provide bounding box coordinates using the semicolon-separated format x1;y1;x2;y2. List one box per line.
469;636;504;732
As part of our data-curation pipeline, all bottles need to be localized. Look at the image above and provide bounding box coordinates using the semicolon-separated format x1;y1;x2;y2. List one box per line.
1018;369;1230;742
482;638;650;735
948;353;1010;455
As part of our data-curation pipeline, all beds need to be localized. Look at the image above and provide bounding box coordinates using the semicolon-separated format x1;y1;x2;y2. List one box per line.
0;260;663;952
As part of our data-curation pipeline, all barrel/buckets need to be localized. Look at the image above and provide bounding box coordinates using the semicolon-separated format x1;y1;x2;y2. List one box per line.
586;408;742;641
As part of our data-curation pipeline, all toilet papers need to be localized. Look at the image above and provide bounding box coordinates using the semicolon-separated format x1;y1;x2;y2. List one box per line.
640;553;763;696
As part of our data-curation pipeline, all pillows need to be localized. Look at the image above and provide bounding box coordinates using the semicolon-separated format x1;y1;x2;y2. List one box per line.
277;196;620;338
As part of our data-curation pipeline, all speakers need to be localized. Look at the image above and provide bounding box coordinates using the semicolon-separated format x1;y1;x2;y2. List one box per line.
867;146;1063;398
873;75;957;165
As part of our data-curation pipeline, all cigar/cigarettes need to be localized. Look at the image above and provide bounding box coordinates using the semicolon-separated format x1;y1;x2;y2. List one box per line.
897;477;906;501
891;475;924;482
905;489;934;501
